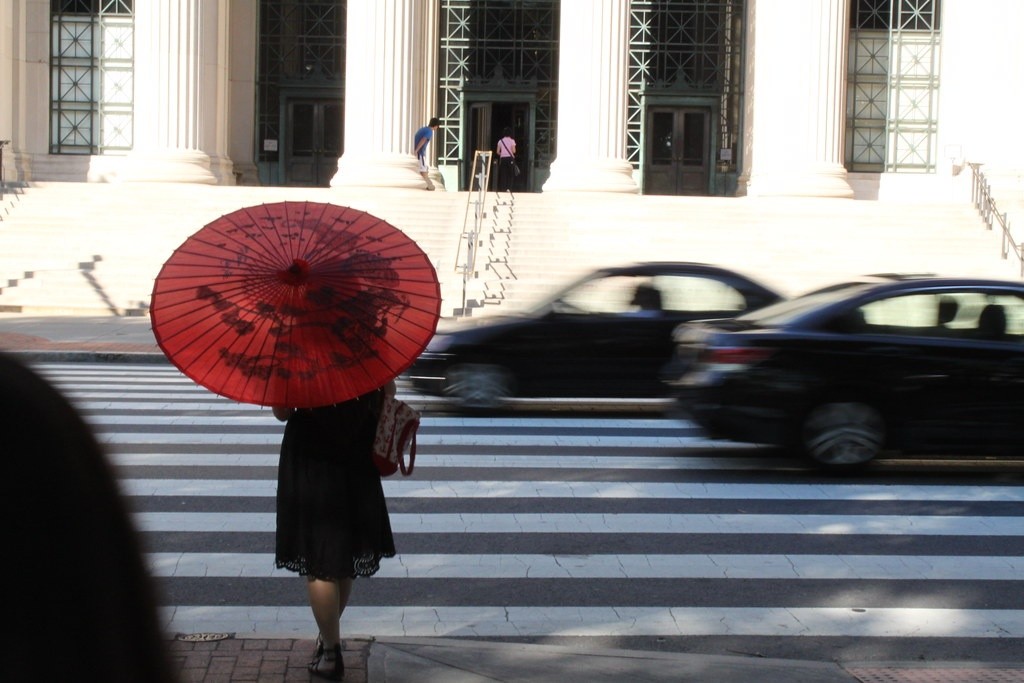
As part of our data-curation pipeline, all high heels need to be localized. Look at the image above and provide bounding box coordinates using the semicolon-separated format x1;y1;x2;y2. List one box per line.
308;643;345;683
314;631;324;659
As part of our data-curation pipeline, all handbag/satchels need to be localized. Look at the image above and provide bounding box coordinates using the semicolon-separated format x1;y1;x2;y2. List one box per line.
370;378;420;478
512;159;521;177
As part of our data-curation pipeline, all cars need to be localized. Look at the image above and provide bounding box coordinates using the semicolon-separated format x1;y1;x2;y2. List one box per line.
665;280;1024;478
400;258;868;418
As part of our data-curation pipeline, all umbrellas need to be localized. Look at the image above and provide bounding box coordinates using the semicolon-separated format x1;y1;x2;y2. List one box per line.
149;201;444;411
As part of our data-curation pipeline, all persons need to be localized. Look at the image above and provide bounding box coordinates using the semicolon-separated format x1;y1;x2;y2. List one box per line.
273;377;396;681
979;305;1006;332
415;118;441;173
631;285;662;309
497;127;516;192
